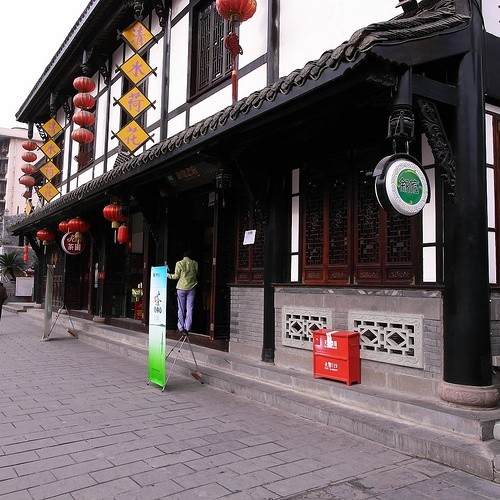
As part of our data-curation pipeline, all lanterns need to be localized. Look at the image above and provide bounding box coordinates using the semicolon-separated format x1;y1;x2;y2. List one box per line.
103;201;128;245
24;246;28;264
37;228;54;255
20;139;37;186
72;77;95;144
68;216;90;239
59;218;71;233
216;0;256;23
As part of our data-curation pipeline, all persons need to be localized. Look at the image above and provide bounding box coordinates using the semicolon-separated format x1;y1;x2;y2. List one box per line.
167;251;199;335
0;282;8;319
